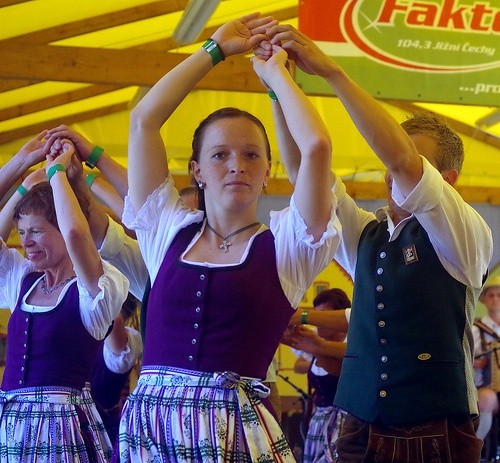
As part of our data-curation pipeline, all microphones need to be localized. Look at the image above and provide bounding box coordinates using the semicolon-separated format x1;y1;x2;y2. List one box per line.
474;319;498;339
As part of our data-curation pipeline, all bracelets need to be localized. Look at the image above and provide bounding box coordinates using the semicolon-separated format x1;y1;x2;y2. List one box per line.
268;91;278;101
17;185;29;197
202;38;225;66
46;164;64;182
84;171;99;189
301;309;308;324
85;145;104;170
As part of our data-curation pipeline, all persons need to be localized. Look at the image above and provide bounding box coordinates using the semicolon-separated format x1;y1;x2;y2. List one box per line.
293;288;352;463
253;24;493;463
0;143;131;463
42;122;198;302
283;307;353;358
88;291;142;447
117;12;343;463
471;276;500;462
0;129;51;203
